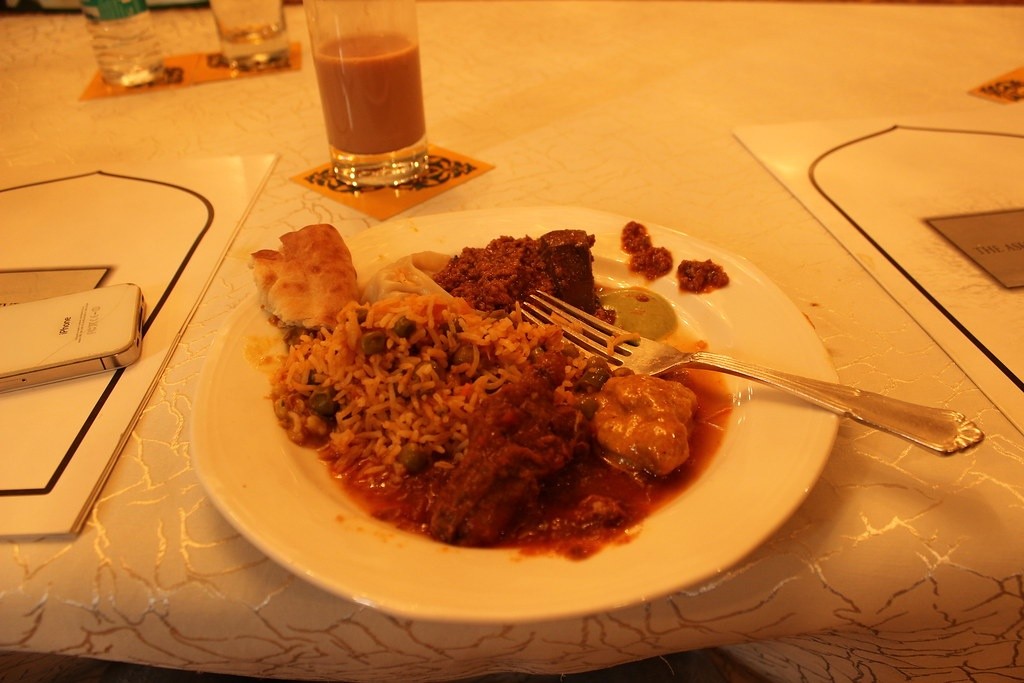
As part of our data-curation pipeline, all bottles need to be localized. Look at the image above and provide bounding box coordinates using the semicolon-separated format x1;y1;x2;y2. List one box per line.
78;0;166;89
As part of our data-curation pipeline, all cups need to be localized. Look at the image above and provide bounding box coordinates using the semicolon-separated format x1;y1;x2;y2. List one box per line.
209;0;295;74
301;0;432;185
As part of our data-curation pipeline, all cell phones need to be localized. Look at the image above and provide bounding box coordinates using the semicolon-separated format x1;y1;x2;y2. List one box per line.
0;283;146;396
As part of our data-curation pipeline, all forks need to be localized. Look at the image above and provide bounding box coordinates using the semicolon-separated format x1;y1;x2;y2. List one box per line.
514;284;986;460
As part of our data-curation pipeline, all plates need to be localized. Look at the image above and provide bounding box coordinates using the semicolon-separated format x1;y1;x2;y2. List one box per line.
192;203;845;622
